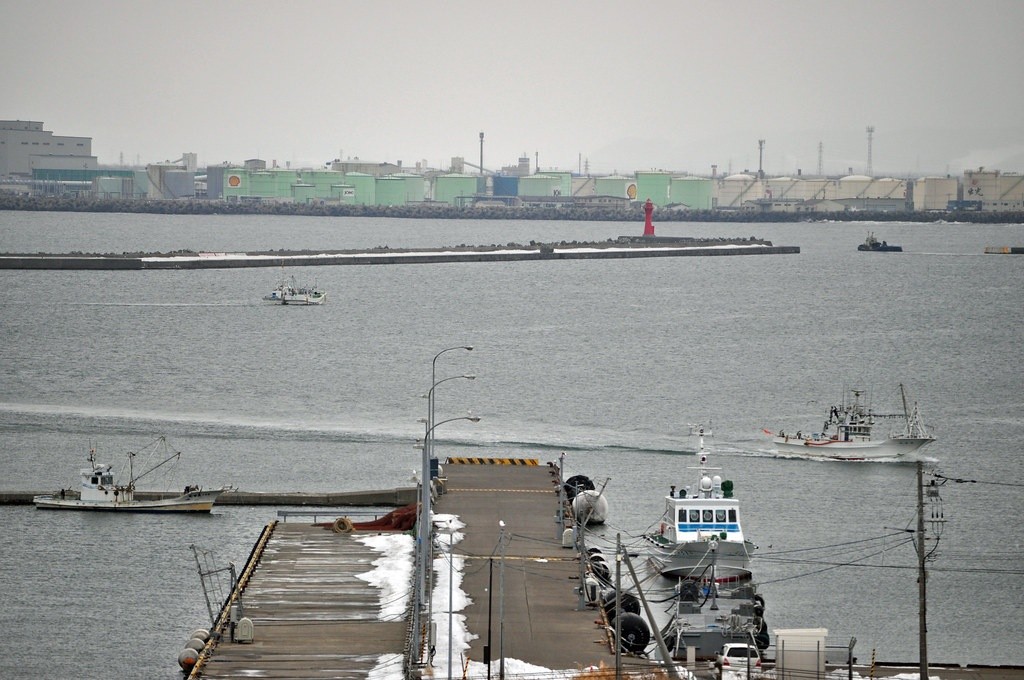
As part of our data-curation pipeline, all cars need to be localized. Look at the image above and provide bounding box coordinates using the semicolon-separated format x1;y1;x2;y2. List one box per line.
713;643;763;675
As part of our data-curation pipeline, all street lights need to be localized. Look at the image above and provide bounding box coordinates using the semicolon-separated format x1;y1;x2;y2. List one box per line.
420;374;478;606
431;345;474;457
411;416;481;661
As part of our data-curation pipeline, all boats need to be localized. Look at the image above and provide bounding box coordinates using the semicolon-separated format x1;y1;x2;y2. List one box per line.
32;435;228;514
263;282;285;301
640;417;755;587
280;278;328;305
858;230;904;252
759;380;939;463
